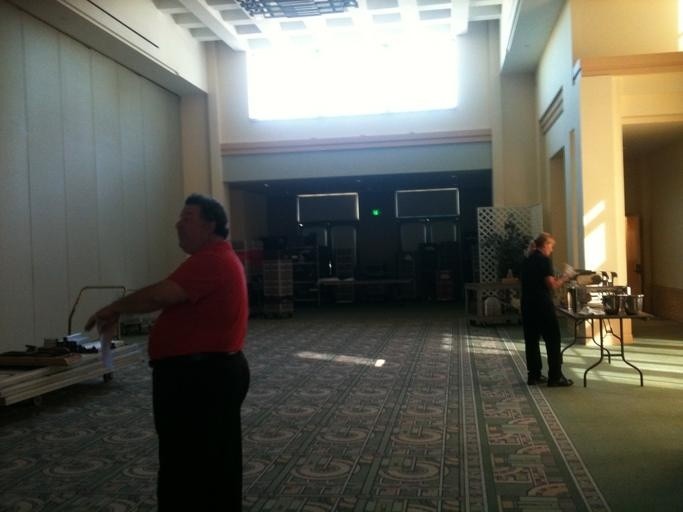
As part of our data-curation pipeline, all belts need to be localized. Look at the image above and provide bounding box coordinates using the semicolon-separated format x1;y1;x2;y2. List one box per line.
148;352;241;369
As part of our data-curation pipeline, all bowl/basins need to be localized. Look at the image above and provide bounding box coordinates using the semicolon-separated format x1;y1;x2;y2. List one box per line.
602;296;624;315
623;294;644;315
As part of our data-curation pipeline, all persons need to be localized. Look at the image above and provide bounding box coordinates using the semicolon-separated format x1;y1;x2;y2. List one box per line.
520;232;574;387
85;192;250;512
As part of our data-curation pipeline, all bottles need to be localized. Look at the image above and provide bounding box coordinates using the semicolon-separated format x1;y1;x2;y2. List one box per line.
567;280;577;314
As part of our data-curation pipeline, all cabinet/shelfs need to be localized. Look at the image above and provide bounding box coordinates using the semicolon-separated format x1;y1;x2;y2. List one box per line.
230;239;462;318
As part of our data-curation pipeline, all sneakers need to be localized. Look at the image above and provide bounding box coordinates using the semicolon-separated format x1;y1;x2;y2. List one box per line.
548;376;573;386
528;373;548;385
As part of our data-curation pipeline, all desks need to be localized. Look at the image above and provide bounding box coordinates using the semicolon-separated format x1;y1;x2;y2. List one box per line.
555;303;655;388
464;281;524;327
